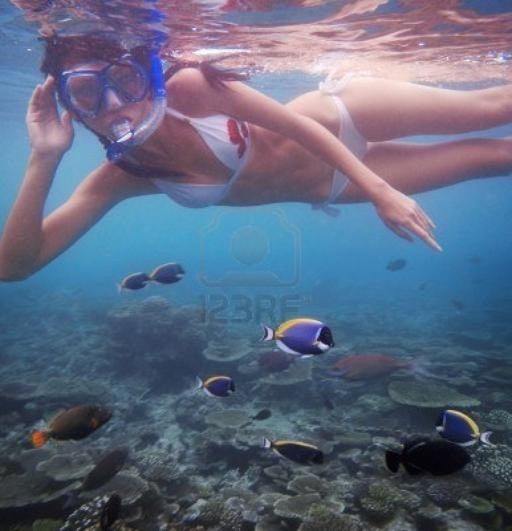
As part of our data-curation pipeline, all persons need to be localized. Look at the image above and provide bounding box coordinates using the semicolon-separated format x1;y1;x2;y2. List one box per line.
0;32;511;282
15;0;512;88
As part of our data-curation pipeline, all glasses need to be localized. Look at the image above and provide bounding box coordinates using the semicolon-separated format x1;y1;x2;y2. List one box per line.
56;55;149;119
